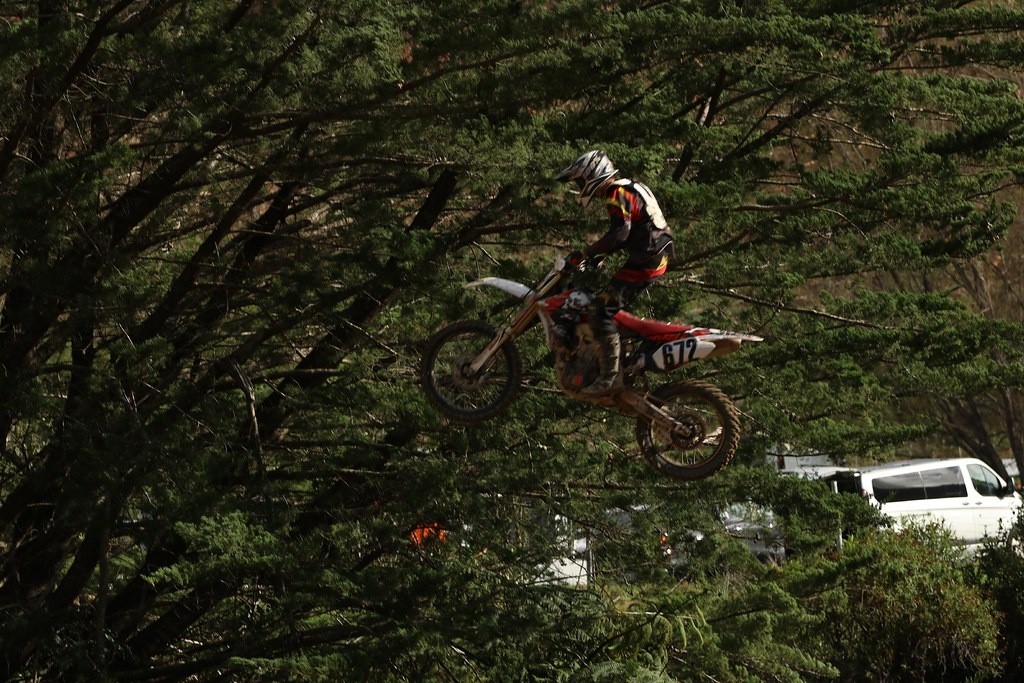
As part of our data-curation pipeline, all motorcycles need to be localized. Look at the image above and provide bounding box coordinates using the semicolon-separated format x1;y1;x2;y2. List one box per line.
419;249;766;482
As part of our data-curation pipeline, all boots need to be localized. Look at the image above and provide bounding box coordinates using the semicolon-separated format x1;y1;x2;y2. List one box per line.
580;333;625;397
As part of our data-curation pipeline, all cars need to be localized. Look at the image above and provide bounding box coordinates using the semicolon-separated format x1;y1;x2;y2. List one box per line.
411;454;855;602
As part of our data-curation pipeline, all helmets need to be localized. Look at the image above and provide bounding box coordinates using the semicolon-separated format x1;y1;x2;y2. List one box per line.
556;150;619;208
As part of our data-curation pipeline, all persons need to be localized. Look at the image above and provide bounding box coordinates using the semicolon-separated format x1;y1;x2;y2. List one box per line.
557;151;675;396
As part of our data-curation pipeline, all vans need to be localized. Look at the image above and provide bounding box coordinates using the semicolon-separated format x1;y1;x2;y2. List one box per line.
836;455;1024;572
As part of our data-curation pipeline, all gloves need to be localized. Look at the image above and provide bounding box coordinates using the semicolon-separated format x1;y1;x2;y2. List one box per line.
561;250;584;278
